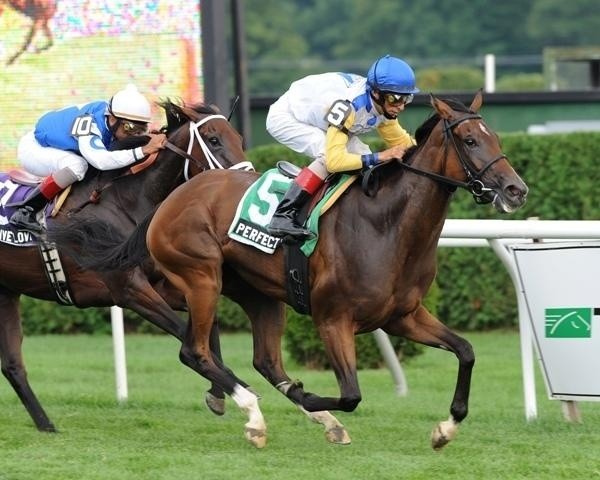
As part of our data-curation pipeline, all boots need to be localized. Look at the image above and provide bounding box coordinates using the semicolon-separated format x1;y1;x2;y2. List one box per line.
7;174;60;233
268;164;323;239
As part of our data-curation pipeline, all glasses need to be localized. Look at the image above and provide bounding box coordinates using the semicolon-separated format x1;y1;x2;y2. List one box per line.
376;94;416;107
120;123;149;136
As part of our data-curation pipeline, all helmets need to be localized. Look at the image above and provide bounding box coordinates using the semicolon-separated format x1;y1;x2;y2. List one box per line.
104;88;153;123
367;54;422;94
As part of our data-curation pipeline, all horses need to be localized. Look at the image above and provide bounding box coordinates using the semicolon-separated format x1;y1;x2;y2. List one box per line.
0;0;58;67
34;88;528;452
0;95;263;434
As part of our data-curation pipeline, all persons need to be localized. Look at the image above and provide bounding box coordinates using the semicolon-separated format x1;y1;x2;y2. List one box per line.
264;54;420;239
9;88;167;231
